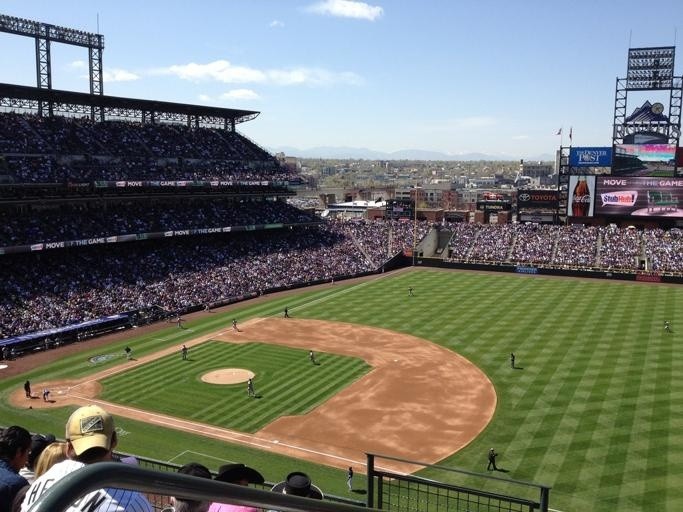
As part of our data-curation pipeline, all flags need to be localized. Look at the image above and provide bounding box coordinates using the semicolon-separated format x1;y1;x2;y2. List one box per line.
555;128;572;138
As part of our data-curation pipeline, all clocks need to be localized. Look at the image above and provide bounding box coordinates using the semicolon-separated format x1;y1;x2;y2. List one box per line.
650;102;663;115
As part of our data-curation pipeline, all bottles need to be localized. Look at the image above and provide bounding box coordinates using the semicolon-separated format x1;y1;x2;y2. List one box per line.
571;175;591;218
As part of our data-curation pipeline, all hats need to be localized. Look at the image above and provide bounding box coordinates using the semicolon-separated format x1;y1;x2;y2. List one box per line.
65;403;115;457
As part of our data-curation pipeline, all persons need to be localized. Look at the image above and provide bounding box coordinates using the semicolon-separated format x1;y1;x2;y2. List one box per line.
486;447;498;471
246;379;255;398
1;111;403;362
208;462;266;512
21;405;153;512
269;471;325;511
345;466;354;491
308;350;315;365
509;353;515;369
169;462;214;512
0;426;71;512
408;286;414;296
664;320;671;332
23;380;31;396
401;217;682;278
42;389;50;401
